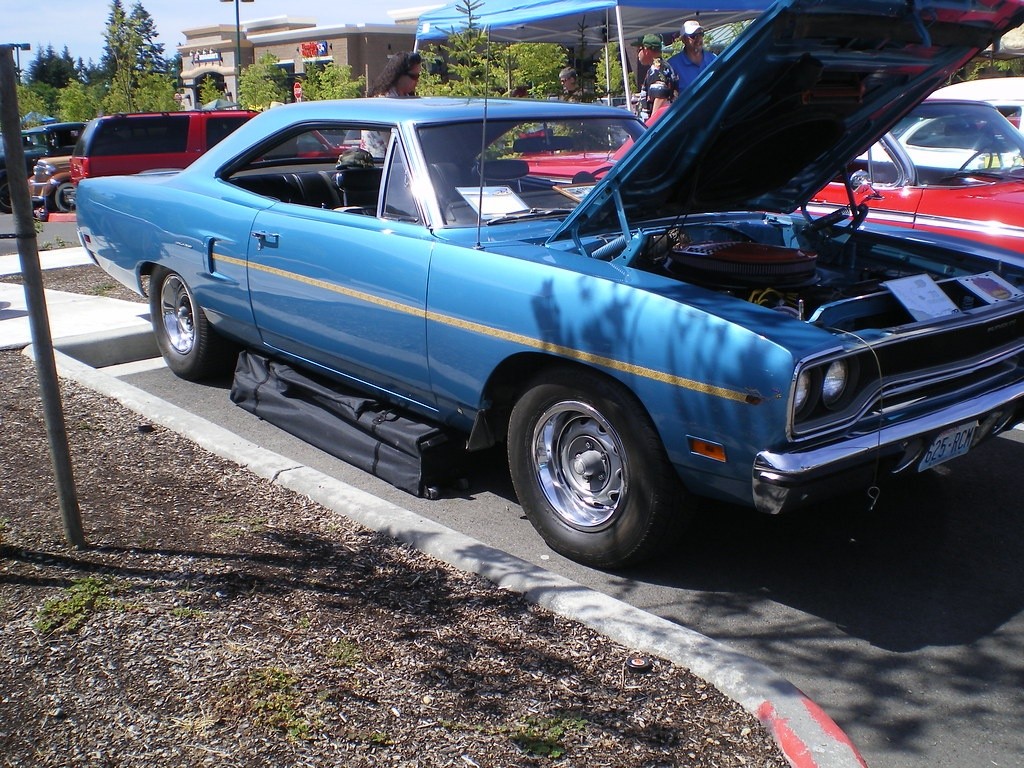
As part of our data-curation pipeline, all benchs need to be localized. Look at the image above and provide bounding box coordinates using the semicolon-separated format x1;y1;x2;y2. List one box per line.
231;169;383;207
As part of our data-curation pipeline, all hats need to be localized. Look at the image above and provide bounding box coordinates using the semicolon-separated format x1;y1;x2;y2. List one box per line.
630;33;662;48
680;20;704;37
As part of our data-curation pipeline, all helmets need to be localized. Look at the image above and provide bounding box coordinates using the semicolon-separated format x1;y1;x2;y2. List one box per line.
335;148;374;170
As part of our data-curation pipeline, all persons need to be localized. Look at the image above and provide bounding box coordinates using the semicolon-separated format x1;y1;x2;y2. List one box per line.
559;66;592;103
359;53;423;159
667;20;718;95
631;34;679;123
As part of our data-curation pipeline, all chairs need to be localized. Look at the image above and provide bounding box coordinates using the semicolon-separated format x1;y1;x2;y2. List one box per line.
476;160;530;193
428;162;464;202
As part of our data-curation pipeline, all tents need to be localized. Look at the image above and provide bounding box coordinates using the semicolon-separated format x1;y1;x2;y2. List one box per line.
413;0;776;108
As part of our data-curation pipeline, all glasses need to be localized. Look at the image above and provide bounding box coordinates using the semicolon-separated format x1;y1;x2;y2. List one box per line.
684;32;704;39
560;78;568;82
403;70;420;80
637;47;644;54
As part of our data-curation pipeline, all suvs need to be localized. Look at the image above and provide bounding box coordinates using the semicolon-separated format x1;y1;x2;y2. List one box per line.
67;108;360;186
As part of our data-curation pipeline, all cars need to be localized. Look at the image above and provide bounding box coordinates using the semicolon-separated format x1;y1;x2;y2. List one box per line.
510;97;1024;257
0;119;89;216
855;75;1024;172
74;0;1024;571
